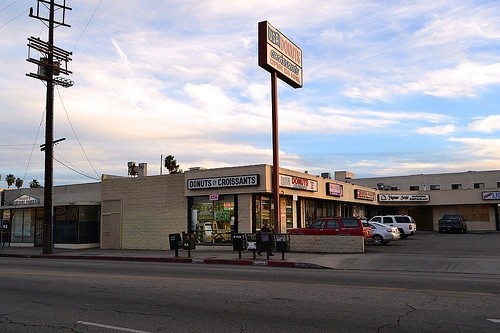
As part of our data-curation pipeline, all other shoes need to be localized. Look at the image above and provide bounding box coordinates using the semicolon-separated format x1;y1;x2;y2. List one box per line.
269;253;274;257
257;252;262;256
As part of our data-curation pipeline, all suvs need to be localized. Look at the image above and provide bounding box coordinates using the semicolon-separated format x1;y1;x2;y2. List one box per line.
370;213;416;240
286;216;373;243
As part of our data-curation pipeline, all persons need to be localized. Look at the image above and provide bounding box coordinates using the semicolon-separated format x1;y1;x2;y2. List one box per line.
257;223;275;256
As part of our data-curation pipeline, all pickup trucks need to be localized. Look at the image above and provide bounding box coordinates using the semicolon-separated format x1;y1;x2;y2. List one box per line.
438;214;467;234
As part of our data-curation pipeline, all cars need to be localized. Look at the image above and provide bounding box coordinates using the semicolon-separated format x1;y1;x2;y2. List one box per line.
360;220;401;247
